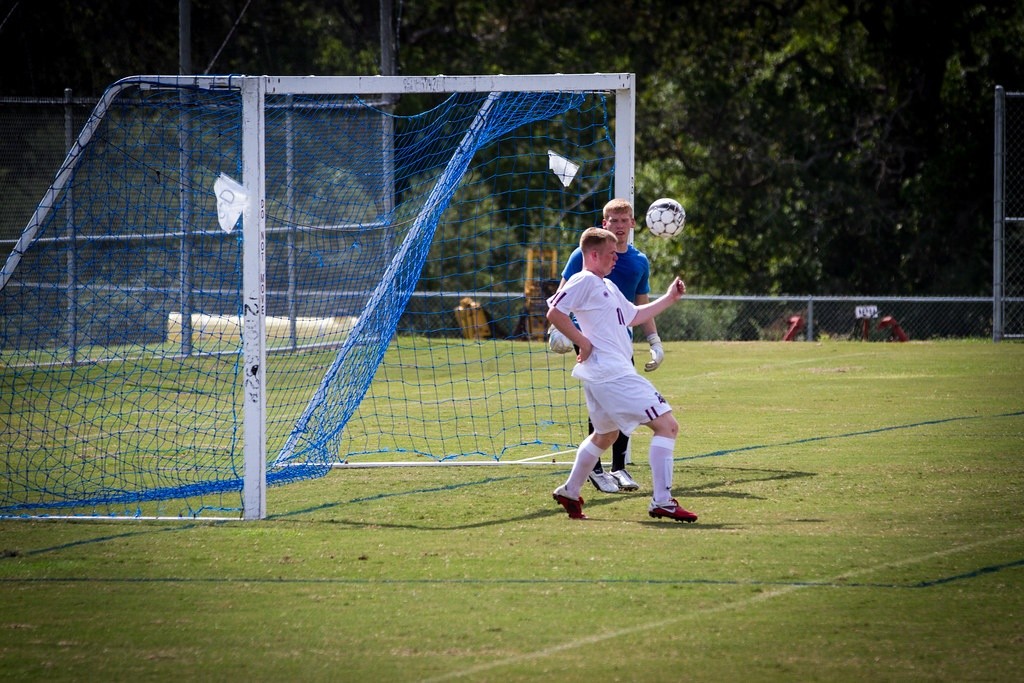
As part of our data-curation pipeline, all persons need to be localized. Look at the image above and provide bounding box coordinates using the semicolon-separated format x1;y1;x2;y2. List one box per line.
549;200;664;493
547;227;698;522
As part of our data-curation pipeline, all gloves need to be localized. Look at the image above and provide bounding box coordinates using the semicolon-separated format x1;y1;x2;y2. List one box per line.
547;324;574;355
644;333;664;372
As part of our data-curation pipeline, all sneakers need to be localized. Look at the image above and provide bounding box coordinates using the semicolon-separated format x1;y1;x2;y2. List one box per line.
648;497;698;524
552;484;589;519
609;467;639;491
587;466;620;493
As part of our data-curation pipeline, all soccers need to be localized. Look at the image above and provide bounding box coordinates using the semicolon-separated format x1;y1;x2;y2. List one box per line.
645;198;686;238
